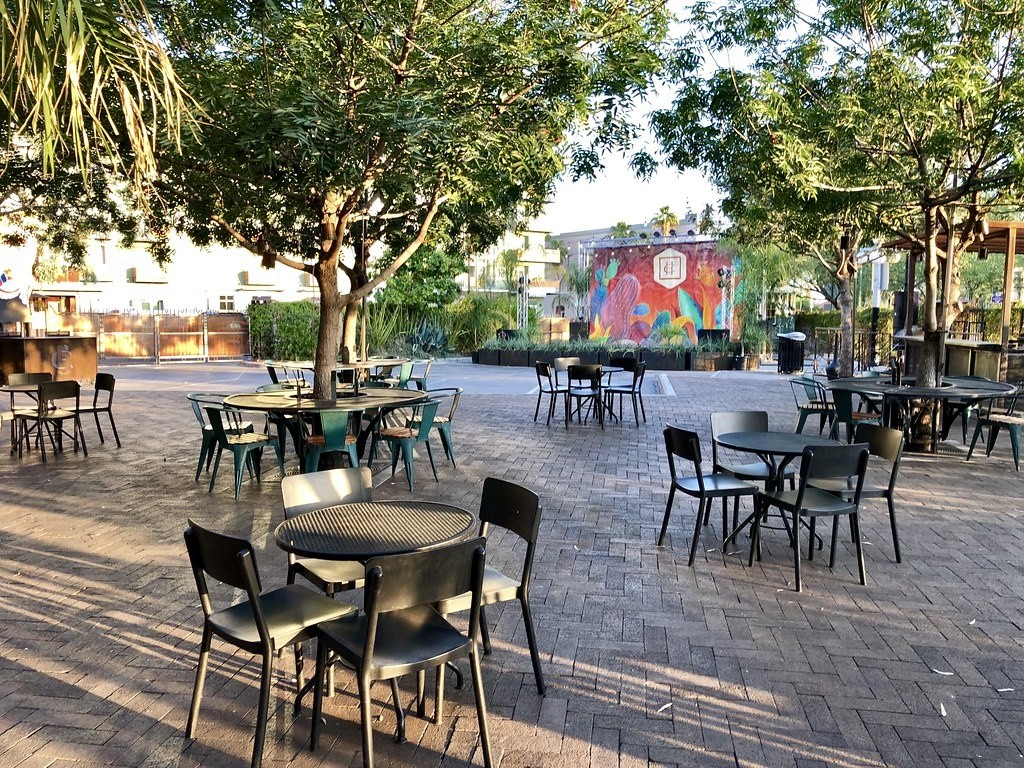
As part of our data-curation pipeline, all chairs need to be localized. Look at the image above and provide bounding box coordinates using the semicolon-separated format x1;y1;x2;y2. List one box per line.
421;477;546;726
658;423;762;567
790;370;1024;471
704;411;796;544
183;518;358;768
281;466;374;697
310;536;493;768
8;371;121;463
791;423;903;564
186;360;465;492
535;356;647;430
749;442;869;592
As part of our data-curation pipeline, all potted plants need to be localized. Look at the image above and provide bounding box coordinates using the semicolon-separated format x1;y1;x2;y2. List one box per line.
470;290;766;371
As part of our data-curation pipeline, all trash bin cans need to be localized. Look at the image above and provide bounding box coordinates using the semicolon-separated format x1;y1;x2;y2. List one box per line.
777;332;806;373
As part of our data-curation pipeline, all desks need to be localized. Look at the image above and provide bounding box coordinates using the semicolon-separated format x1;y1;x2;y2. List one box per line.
271;358;411;369
565;365;625;424
0;384;57;454
274;499;476;746
224;386;430;413
822;375;1018;398
712;430;841;554
